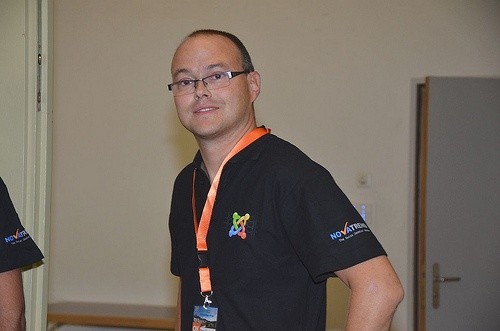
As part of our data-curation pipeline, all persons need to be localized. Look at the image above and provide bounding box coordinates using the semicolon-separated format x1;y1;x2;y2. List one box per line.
167;28;406;331
0;177;45;331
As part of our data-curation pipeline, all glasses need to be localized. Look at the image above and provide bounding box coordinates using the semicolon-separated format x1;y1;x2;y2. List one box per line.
168;70;250;95
